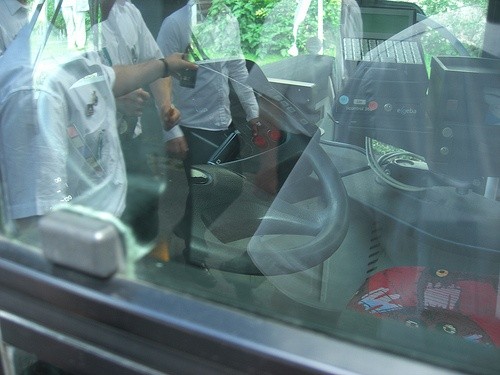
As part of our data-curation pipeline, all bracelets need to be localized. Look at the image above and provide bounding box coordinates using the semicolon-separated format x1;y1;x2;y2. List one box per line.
160;59;170;78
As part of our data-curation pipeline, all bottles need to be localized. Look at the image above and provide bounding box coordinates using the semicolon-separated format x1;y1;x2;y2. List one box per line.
179;30;199;89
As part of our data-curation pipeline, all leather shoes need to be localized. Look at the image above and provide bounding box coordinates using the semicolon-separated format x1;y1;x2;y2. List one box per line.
173;217;209;256
186;258;217;288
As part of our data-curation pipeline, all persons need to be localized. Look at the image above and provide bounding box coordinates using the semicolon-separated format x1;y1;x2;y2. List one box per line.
246;0;499;311
244;0;370;202
1;0;200;262
155;0;259;270
